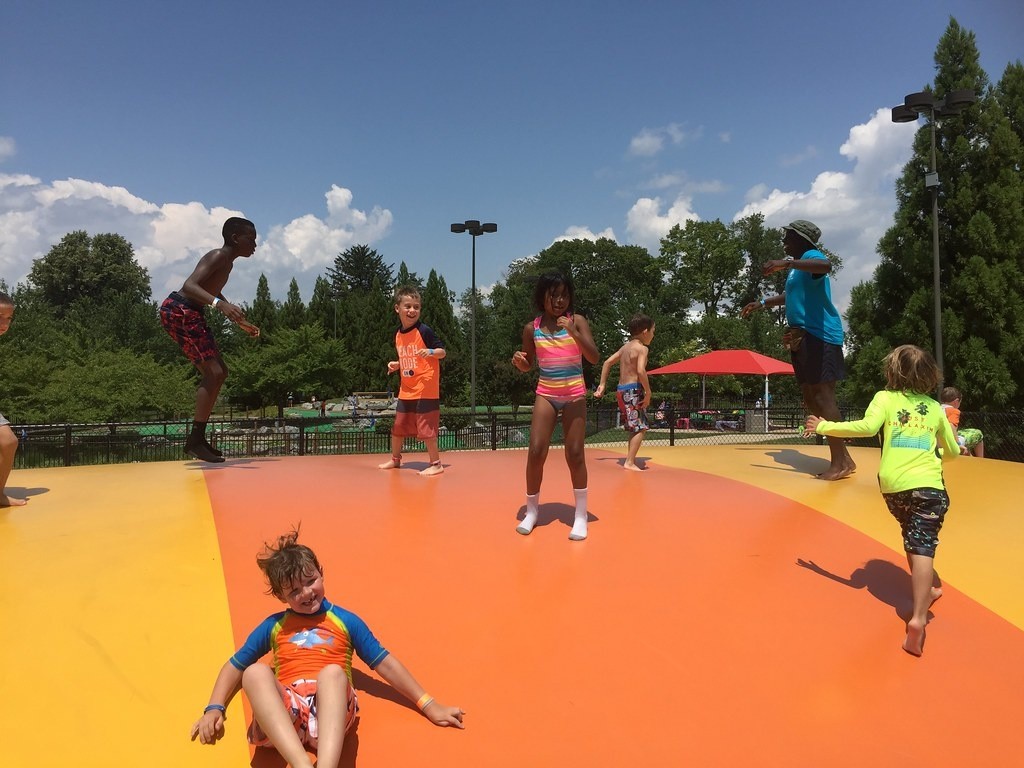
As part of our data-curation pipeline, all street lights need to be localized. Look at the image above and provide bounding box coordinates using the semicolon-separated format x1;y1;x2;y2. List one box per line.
450;220;498;433
891;89;976;402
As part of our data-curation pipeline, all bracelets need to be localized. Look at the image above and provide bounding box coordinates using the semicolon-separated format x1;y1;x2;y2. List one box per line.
760;300;766;308
429;349;433;355
204;704;226;715
784;257;794;270
416;694;434;712
211;297;220;308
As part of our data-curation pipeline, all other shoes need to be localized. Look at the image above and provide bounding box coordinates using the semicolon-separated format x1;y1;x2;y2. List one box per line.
184;438;225;462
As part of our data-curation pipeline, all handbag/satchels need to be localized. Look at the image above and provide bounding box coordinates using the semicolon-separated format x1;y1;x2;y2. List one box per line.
782;327;809;352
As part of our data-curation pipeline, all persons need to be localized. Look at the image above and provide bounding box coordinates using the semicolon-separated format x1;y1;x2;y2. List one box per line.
754;388;773;413
594;312;656;471
190;518;466;768
740;220;857;479
160;217;260;463
803;345;962;656
511;266;599;540
287;391;375;428
938;387;984;458
0;291;28;506
378;284;445;476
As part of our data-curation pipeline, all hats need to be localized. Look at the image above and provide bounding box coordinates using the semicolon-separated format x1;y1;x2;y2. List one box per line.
780;220;822;250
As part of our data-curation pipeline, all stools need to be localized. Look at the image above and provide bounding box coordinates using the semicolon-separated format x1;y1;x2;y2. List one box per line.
677;418;690;430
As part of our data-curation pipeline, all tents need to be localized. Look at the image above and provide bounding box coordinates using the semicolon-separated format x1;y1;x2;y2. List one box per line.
648;349;795;434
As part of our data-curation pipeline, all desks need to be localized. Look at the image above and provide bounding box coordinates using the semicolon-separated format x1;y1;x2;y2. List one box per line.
768;411;802;429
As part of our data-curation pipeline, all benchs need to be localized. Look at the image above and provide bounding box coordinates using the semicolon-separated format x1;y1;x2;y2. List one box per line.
768;417;805;429
